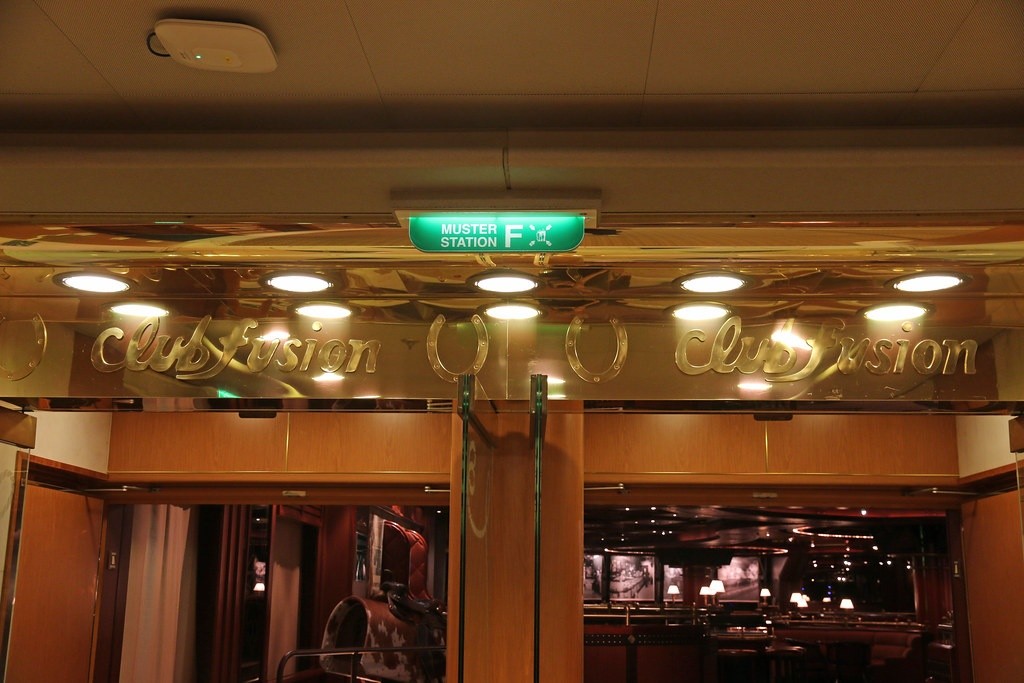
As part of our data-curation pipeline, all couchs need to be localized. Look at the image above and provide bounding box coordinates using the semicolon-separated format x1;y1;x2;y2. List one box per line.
772;629;924;683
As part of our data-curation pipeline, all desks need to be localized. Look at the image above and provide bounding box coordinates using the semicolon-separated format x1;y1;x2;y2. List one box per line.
710;632;773;683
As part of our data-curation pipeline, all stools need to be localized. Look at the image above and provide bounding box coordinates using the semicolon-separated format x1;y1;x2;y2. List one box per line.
719;649;759;683
765;646;808;683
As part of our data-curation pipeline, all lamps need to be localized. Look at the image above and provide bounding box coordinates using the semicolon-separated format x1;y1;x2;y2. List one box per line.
856;301;938;325
698;586;713;607
710;579;726;608
464;266;547;295
882;271;970;294
286;298;366;323
104;301;174;321
661;300;733;322
671;269;756;295
51;265;137;295
257;268;336;295
475;299;548;324
839;598;854;621
789;592;811;614
666;584;680;608
759;588;771;606
